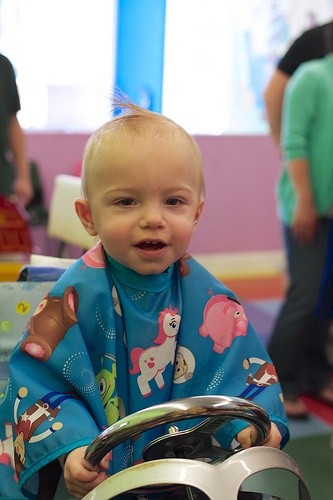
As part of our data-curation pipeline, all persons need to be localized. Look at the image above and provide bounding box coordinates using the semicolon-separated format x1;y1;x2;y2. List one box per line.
266;18;333;418
0;53;33;207
0;103;290;500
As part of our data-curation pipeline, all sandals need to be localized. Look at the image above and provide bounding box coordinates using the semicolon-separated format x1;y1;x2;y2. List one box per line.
315;388;333;406
282;397;309;417
